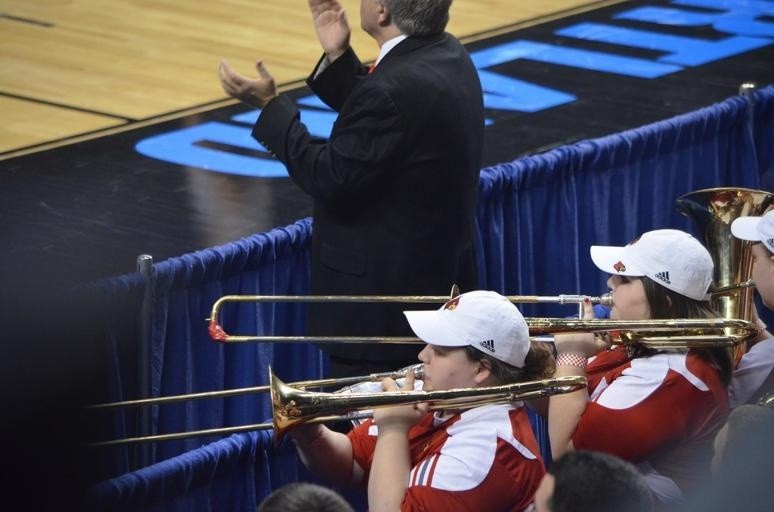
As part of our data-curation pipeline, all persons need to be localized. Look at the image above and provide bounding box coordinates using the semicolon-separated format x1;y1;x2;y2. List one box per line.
219;0;485;391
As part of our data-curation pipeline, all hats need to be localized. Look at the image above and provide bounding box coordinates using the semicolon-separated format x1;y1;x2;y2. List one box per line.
730;211;774;254
589;229;715;305
402;289;531;369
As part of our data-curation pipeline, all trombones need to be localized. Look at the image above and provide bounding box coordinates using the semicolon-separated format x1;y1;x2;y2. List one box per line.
87;363;589;453
210;291;757;354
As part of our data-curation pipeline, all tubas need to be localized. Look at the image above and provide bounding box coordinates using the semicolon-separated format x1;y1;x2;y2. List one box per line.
673;187;774;408
